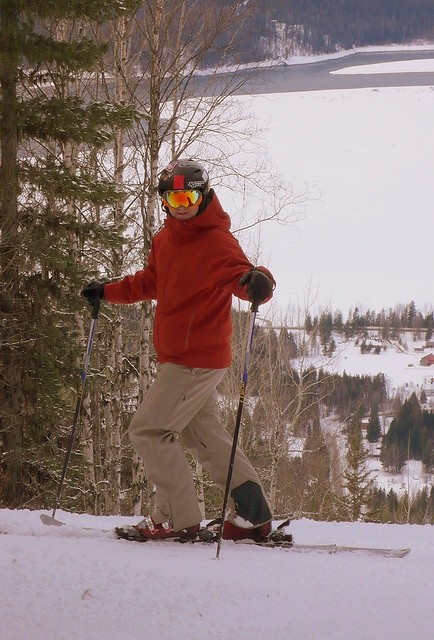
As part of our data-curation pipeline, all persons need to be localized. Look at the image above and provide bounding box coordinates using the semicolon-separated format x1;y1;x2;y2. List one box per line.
79;158;293;550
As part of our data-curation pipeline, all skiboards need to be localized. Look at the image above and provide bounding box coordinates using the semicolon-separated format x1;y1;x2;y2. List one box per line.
40;514;410;559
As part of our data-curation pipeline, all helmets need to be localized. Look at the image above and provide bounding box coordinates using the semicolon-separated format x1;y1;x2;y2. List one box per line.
157;159;209;212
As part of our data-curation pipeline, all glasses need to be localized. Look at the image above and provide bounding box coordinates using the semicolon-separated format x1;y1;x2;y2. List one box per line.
161;190;202;208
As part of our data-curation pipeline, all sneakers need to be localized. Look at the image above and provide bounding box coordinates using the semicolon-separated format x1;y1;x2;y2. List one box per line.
134;515;200;539
206;511;273;542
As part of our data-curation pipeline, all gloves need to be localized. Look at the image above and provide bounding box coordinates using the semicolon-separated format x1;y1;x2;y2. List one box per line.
83;281;104;304
239;270;272;301
